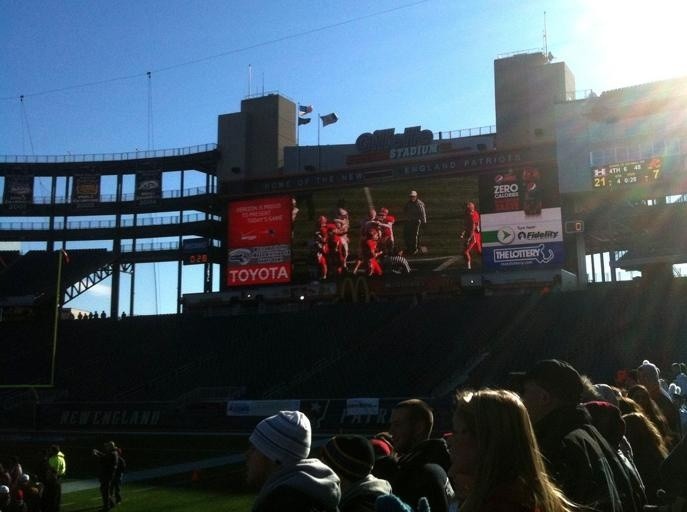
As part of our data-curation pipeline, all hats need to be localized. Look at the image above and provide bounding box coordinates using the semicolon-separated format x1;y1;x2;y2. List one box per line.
410;190;417;197
321;435;375;483
509;360;583;395
248;409;312;466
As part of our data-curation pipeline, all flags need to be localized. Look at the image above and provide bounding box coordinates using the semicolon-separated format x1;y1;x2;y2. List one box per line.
300;106;312;113
320;113;338;127
298;117;311;126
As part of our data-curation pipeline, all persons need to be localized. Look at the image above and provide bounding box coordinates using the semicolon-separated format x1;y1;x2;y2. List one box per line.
397;190;426;256
460;203;482;270
246;360;686;511
77;311;127;320
2;441;66;509
313;207;411;279
92;441;126;509
